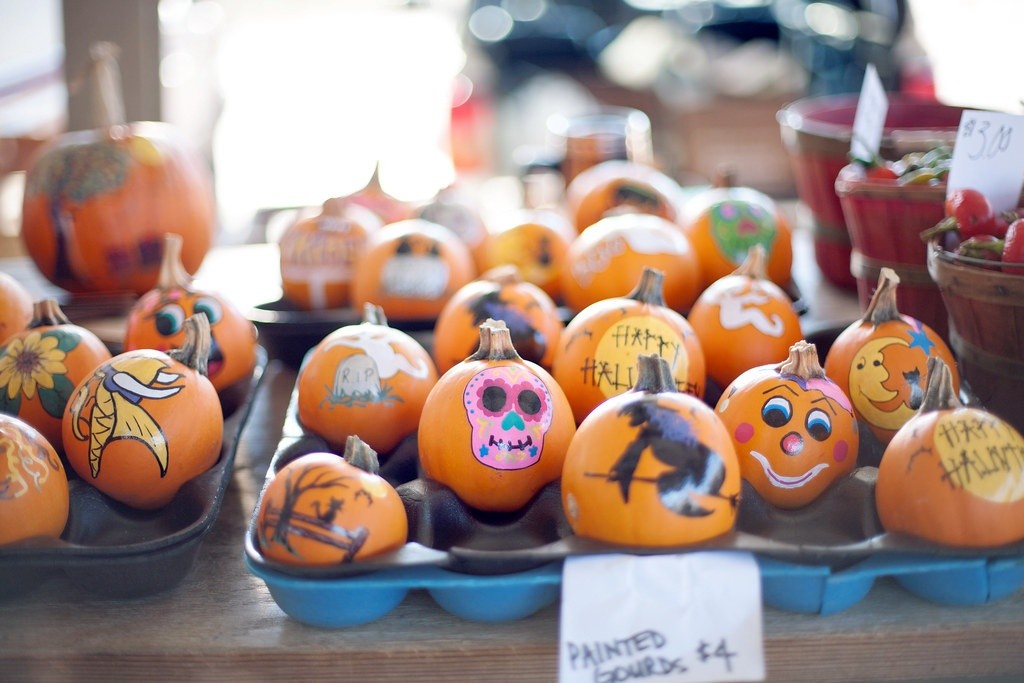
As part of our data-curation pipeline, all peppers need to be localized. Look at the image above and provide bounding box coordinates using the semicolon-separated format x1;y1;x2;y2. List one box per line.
846;144;1024;276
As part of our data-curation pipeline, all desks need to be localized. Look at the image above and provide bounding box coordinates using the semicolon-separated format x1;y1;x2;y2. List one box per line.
0;358;1024;683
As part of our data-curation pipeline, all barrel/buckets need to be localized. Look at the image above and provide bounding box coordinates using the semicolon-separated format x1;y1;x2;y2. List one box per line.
776;90;978;293
924;234;1024;436
836;161;956;359
